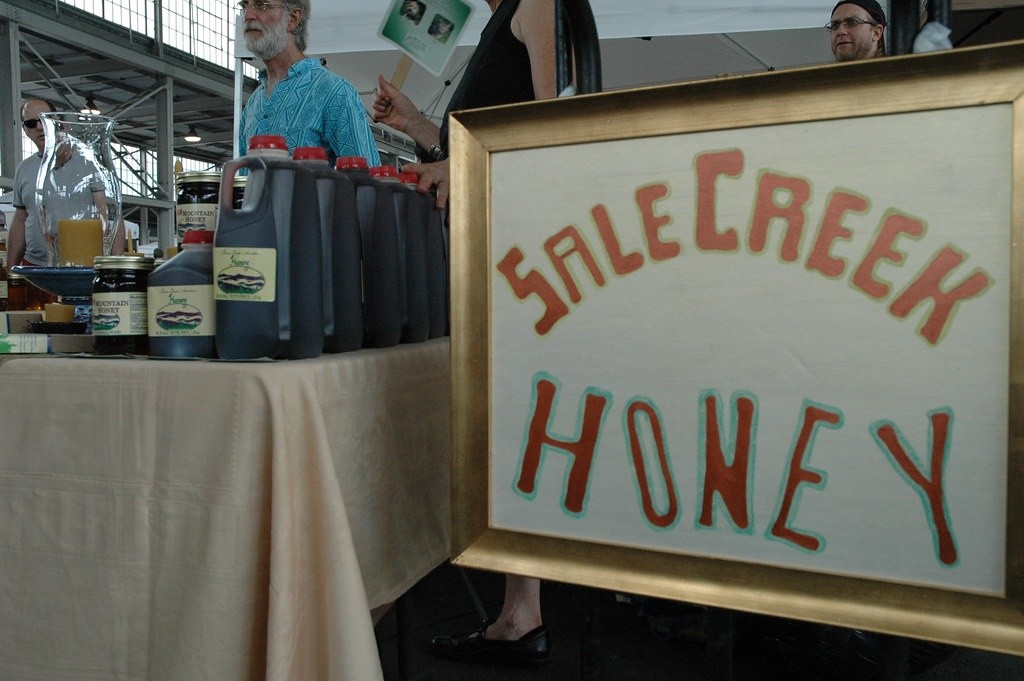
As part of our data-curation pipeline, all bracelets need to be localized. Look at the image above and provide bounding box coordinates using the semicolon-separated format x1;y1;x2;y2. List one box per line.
427;144;442;162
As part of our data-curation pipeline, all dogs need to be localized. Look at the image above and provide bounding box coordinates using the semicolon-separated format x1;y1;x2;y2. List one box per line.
428;18;452;38
402;1;420;24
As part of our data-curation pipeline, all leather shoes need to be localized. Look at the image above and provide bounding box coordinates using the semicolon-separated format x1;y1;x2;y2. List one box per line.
426;624;553;667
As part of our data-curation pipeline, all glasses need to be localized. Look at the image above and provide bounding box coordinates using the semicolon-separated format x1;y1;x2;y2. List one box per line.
824;17;876;30
235;0;287;13
21;117;50;127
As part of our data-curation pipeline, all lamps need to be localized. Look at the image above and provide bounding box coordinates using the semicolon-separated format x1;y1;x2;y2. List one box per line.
183;126;201;142
81;95;101;116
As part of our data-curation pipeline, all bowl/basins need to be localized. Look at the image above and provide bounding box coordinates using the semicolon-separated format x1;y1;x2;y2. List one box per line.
11;266;93;296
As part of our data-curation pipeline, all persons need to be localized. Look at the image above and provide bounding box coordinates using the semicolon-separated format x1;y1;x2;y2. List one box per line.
239;0;382;177
825;0;885;62
372;0;603;660
7;97;125;272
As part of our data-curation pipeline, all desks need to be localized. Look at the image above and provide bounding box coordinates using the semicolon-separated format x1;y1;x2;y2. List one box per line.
0;335;452;681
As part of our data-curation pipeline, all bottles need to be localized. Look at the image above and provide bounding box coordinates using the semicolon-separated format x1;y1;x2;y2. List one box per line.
211;134;453;361
22;110;122;268
146;229;216;360
0;257;10;312
